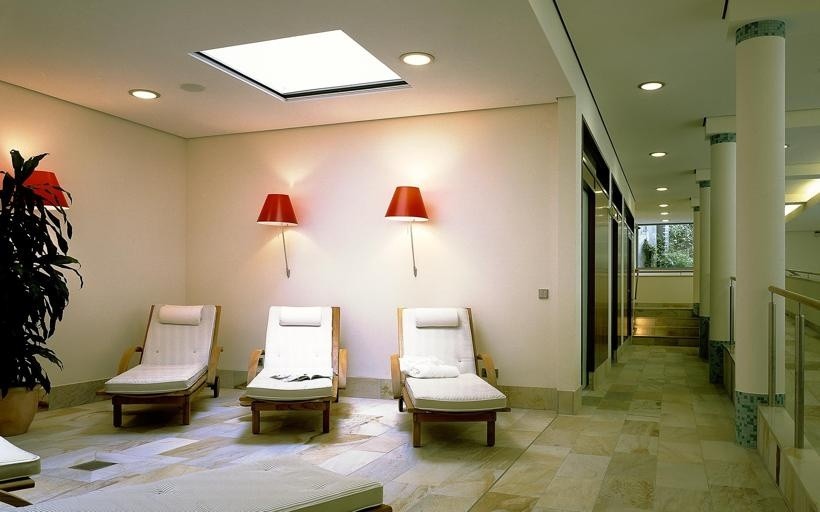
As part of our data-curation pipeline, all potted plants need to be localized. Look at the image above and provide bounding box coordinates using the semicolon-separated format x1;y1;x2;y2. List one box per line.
0;150;83;436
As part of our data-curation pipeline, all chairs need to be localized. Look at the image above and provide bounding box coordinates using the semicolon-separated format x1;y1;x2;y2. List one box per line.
0;436;41;491
239;306;347;434
96;305;223;427
391;308;511;448
0;457;392;512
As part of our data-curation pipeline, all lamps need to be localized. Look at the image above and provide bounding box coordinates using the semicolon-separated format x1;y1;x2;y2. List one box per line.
257;194;298;278
22;171;69;210
385;187;429;277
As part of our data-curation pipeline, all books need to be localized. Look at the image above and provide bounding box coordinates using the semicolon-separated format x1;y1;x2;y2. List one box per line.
270;369;331;382
